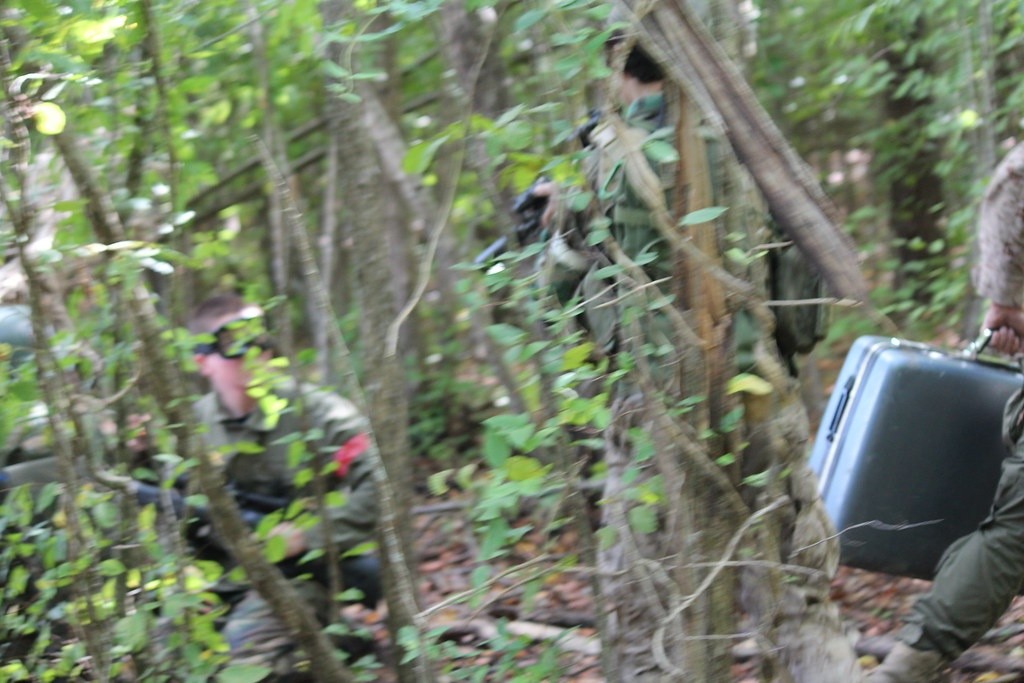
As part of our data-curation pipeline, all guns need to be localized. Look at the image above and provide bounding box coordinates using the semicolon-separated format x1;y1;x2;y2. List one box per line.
473;105;606;268
97;453;297;588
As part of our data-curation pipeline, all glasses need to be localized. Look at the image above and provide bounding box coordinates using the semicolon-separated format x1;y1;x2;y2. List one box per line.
216;316;271;359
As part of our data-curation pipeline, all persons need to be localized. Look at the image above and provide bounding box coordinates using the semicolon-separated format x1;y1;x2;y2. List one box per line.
538;27;864;659
188;293;394;683
859;140;1024;683
0;304;153;683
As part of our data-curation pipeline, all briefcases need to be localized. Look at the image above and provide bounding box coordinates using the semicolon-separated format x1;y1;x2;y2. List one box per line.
805;324;1022;582
